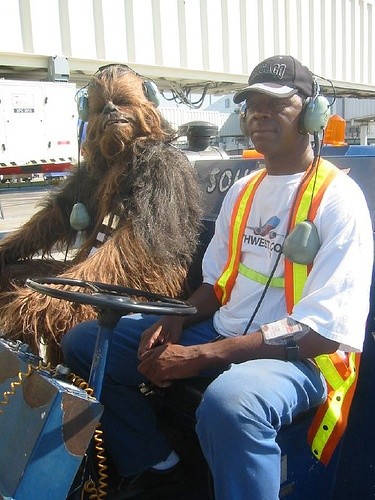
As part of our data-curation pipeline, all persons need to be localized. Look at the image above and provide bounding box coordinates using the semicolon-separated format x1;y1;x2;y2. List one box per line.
61;54;375;500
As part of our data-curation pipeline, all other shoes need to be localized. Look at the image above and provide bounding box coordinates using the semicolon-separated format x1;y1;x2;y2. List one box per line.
115;459;192;499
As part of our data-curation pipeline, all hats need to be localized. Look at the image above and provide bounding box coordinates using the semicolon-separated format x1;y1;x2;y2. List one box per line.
233;55;317;104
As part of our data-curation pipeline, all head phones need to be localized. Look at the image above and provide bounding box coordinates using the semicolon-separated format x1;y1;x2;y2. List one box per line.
74;64;160;122
240;72;336;138
